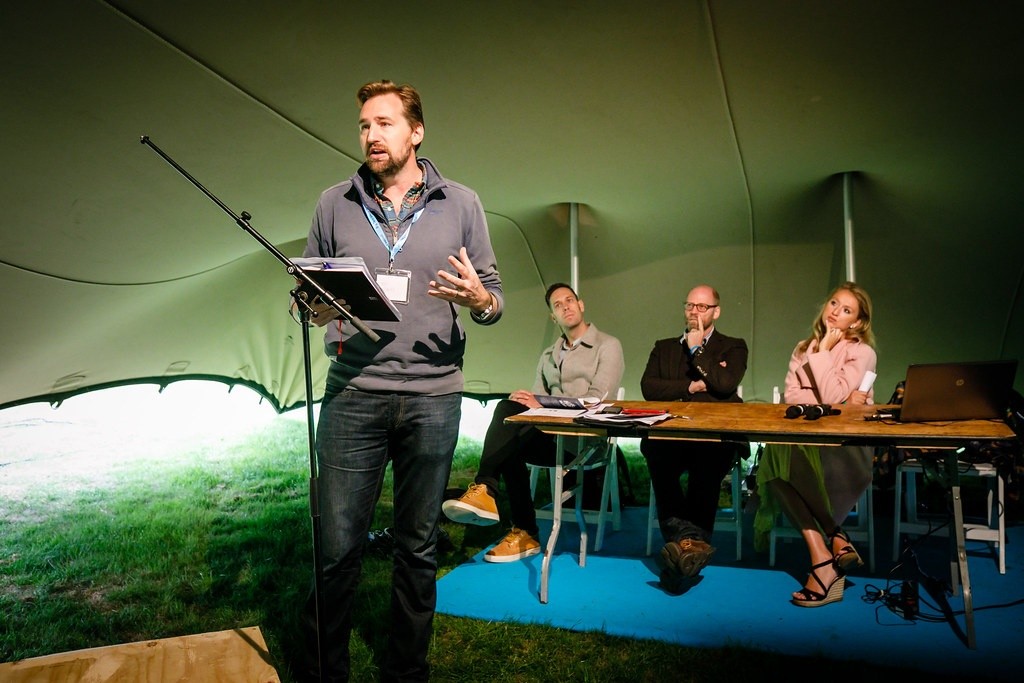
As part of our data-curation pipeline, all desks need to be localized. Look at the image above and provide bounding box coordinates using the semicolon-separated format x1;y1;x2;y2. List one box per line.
503;400;1016;647
1;625;283;683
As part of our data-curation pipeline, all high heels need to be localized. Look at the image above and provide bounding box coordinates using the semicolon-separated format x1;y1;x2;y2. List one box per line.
793;558;845;607
831;535;865;576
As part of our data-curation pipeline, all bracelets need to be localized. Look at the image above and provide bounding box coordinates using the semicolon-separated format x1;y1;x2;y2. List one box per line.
690;346;702;352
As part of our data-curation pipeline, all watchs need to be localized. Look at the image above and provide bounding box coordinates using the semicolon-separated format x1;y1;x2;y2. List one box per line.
692;347;705;358
480;293;494;318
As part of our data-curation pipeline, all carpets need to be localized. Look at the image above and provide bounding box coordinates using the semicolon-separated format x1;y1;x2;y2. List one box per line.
435;501;1024;683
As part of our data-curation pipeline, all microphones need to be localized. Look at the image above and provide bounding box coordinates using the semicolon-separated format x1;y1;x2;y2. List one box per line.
786;405;832;420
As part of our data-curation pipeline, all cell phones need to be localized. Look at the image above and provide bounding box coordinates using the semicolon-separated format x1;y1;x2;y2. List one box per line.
602;406;623;414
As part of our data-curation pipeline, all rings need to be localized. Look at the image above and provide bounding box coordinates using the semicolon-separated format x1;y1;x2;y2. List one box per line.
455;293;459;298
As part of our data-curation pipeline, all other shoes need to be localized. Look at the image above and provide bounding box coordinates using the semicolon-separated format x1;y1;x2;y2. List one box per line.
659;542;683;594
679;537;718;576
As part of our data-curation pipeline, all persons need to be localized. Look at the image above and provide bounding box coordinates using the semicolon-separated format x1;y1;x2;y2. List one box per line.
293;79;505;683
754;282;877;608
641;285;750;593
441;283;626;563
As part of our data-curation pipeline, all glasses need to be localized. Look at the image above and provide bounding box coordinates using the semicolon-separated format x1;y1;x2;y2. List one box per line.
684;302;719;312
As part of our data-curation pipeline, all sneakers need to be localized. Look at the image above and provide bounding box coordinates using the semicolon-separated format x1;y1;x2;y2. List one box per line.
443;482;500;527
484;526;541;563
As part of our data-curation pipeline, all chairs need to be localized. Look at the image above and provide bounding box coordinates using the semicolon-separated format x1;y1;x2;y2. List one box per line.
647;383;743;560
769;385;877;573
893;459;1006;575
526;387;622;551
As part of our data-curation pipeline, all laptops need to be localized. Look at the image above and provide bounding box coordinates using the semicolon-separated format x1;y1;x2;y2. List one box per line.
878;359;1017;421
